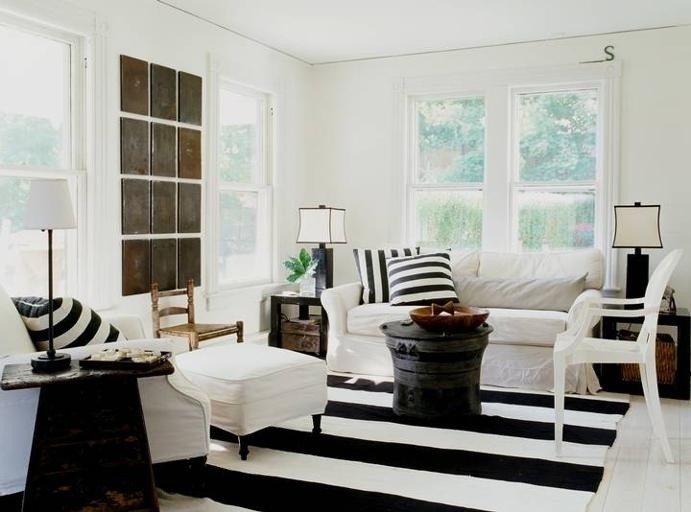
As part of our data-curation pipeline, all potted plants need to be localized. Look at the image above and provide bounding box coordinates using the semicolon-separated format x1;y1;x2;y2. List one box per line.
283;248;314;292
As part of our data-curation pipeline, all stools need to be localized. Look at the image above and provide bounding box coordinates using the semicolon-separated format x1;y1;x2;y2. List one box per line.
174;342;328;461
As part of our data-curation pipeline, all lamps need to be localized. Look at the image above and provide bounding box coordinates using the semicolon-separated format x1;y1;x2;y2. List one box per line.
611;201;663;307
296;205;346;291
21;176;76;373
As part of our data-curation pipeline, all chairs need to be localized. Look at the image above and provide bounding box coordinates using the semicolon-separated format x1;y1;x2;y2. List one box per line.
151;278;244;352
0;284;211;495
552;250;681;463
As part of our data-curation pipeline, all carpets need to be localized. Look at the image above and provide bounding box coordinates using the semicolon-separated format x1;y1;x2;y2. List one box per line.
149;388;630;511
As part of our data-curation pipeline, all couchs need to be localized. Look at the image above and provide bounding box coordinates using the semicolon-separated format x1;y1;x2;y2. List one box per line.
321;248;606;400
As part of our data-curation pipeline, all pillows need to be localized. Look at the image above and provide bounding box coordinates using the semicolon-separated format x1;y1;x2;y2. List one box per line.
11;295;126;351
353;248;419;304
452;271;588;312
385;248;460;306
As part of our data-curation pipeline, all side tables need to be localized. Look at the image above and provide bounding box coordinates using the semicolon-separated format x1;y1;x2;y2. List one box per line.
1;352;174;511
600;307;691;403
268;291;328;364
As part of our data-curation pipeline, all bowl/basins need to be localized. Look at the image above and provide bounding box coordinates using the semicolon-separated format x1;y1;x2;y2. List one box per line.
408;305;490;334
